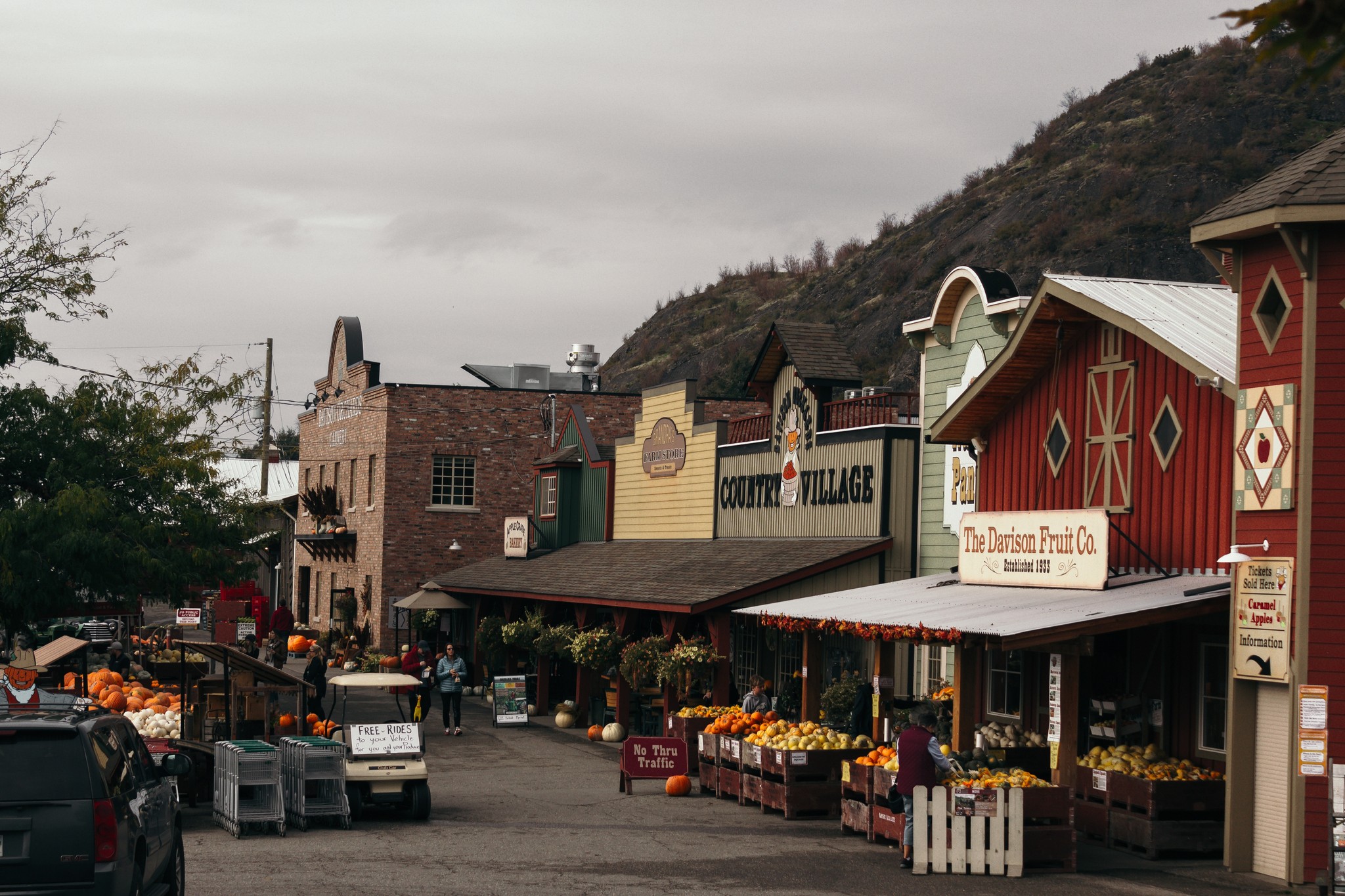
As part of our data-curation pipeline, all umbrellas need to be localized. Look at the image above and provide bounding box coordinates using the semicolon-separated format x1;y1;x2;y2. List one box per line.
390;582;472;610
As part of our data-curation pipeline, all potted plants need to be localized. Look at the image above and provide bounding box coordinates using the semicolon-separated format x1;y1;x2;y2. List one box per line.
298;481;337;534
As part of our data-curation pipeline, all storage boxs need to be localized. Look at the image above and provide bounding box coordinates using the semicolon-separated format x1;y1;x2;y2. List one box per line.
202;580;271;648
1092;699;1103;709
1102;695;1144;711
1090;720;1141;737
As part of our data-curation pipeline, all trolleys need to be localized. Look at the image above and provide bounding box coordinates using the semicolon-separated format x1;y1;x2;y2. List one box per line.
213;736;354;840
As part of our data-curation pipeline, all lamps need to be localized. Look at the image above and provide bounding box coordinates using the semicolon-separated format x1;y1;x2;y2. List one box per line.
321;384;345;403
313;389;336;406
1217;540;1269;563
449;539;463;550
335;380;359;398
275;562;288;569
304;393;322;411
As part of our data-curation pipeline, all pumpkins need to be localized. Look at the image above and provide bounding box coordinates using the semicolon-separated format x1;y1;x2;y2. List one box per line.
855;744;899;771
288;621;318;653
669;705;742;718
436;653;445;658
379;644;409;668
587;724;604;741
329;656;358;671
564;699;575;707
894;687;954;742
350;634;355;640
938;743;1055;790
743;719;876;751
462;681;494;705
1075;742;1226;780
793;670;802;678
665;775;691;796
555;711;575;728
704;710;779;739
528;704;538;716
269;709;341;738
972;721;1047;747
819;710;826;719
763;680;774;690
346;636;348;638
602;723;625;742
57;632;205;739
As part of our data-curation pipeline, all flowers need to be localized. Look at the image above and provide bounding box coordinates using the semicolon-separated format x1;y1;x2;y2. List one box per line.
406;608;441;631
475;605;591;667
334;590;357;622
564;621;727;701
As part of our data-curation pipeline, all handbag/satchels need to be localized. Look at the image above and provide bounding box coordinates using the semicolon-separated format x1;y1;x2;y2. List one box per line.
267;661;274;667
888;784;904;814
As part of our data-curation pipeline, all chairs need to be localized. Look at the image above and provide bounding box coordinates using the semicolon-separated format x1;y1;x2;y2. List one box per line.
601;675;705;737
333;628;364;670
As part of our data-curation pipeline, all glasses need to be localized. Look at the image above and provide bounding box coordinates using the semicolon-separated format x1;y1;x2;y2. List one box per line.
447;648;454;651
309;650;315;653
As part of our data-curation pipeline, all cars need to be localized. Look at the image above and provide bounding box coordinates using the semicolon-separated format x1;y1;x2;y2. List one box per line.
0;616;126;666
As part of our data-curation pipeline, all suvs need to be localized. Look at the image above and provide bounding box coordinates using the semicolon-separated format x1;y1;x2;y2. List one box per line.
0;703;194;896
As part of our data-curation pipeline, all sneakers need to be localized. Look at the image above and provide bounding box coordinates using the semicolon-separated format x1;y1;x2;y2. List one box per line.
445;727;451;735
454;727;462;736
900;856;913;868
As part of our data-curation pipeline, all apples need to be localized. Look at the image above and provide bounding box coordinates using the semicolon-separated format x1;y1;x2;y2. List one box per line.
1094;719;1136;728
1093;692;1135;702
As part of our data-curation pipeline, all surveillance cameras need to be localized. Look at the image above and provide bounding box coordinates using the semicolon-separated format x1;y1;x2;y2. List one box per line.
1194;375;1210;386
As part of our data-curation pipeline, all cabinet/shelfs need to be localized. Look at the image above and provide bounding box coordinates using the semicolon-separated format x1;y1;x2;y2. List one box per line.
1088;694;1148;753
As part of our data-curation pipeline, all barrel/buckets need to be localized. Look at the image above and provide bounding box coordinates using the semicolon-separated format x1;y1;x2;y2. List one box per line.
326;522;331;530
321;524;327;529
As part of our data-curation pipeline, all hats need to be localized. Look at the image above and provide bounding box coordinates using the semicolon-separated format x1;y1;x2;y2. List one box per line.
108;642;122;650
418;640;430;651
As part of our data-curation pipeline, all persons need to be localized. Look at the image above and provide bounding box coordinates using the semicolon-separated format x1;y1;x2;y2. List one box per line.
402;640;435;723
302;652;312;725
436;643;468;735
269;599;294;665
704;661;739;708
237;634;260;660
107;642;131;683
264;628;287;670
741;675;772;714
511;690;516;705
306;644;328;726
895;710;957;870
